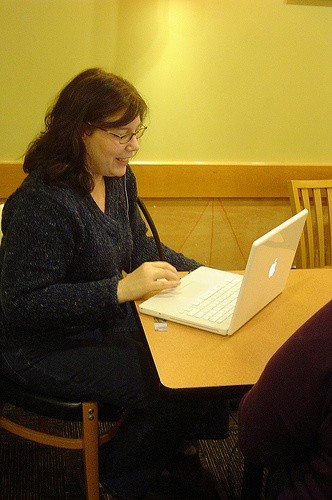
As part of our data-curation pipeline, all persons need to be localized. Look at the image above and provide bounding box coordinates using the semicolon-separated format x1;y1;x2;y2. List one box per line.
0;67;228;500
237;296;331;500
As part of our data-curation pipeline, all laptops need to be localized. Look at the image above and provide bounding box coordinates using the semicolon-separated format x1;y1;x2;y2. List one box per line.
139;208;308;336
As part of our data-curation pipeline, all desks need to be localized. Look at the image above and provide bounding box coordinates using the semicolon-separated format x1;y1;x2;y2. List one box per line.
133;269;332;389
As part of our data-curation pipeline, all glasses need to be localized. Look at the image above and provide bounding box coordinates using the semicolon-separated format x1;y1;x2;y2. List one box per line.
87;122;147;144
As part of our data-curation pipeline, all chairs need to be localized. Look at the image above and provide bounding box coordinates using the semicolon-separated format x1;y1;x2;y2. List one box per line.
288;179;332;269
0;373;134;500
